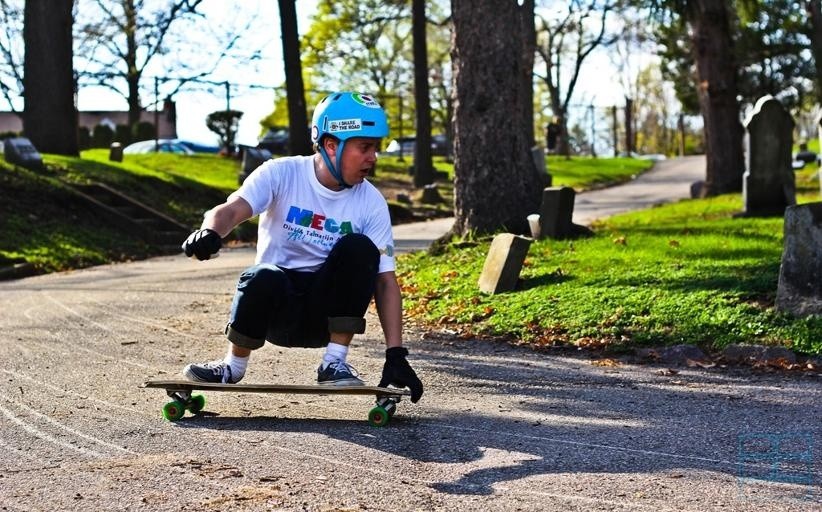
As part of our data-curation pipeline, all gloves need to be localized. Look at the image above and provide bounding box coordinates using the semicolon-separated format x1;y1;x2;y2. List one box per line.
375;345;425;404
180;228;223;261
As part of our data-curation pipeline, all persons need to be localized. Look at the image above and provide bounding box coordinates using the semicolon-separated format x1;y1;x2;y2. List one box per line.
182;90;424;405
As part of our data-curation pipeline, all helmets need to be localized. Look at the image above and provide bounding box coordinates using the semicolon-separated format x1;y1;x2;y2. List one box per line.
310;89;390;143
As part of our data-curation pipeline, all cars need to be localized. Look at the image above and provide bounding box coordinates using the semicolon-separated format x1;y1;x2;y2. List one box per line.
121;140;195;155
258;124;315;157
385;134;447;156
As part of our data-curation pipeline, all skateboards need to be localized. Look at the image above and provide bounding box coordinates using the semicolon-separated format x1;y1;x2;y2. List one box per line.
138;382;412;428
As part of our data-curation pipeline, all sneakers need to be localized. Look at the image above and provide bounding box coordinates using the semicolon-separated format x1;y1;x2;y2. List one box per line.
182;359;245;384
316;359;365;387
